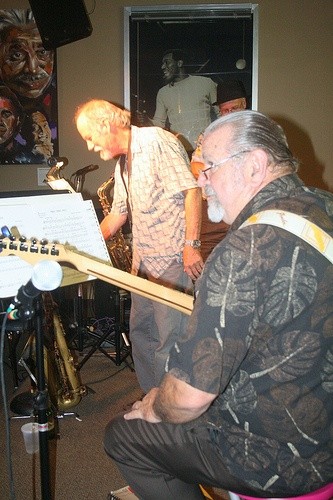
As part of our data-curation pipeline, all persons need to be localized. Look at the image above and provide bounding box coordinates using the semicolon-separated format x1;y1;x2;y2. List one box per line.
212;80;251;116
138;50;221;160
74;100;203;395
102;110;333;500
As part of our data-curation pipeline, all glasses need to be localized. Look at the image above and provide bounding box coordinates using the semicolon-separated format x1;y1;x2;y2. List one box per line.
195;150;254;180
218;101;243;116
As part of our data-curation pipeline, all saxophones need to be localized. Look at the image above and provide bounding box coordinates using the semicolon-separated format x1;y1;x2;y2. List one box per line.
16;290;87;412
41;154;133;275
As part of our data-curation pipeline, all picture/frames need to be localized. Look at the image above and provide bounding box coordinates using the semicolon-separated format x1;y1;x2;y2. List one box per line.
122;3;259;160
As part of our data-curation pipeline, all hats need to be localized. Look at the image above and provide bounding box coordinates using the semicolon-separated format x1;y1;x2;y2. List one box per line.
211;79;246;106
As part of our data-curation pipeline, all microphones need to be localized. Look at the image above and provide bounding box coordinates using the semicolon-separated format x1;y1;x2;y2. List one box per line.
7;259;63;315
74;164;98;177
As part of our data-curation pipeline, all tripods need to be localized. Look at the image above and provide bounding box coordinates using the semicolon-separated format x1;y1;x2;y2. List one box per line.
74;287;135;373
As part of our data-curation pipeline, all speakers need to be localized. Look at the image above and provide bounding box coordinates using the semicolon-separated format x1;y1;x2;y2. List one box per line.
28;0;93;49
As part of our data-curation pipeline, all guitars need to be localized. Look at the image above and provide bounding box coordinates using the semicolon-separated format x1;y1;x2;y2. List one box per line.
0;224;195;316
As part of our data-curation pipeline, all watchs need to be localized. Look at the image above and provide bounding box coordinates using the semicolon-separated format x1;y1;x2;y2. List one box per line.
185;240;201;248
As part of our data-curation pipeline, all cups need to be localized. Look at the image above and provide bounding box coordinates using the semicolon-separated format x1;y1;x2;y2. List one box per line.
21;422;39;454
